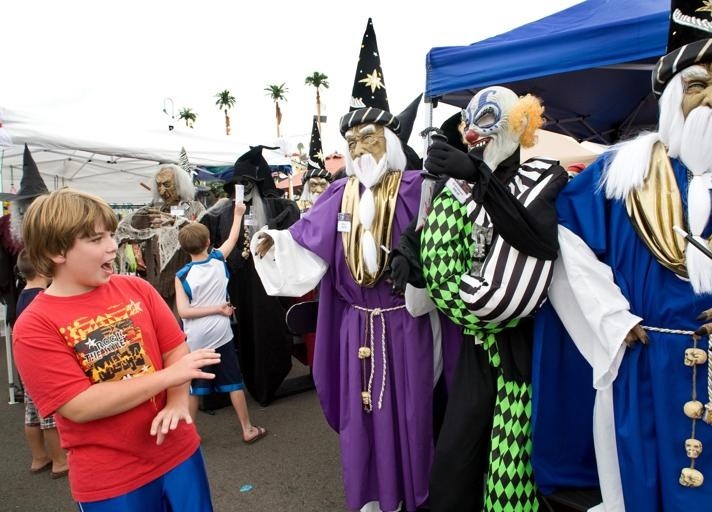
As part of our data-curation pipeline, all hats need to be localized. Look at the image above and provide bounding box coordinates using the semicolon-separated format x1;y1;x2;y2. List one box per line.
301;116;335;182
221;145;280;191
339;16;402;138
650;0;711;98
1;142;53;204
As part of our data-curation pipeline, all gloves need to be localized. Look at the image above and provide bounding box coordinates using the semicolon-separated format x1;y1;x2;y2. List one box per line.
389;255;410;293
424;138;479;184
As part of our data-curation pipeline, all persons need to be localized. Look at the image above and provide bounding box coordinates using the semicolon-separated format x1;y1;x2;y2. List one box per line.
116;160;332;444
248;64;712;510
15;247;71;479
14;190;221;507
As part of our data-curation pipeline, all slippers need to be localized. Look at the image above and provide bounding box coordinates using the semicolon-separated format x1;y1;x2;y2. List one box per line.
50;464;70;479
32;453;51;474
245;425;266;444
